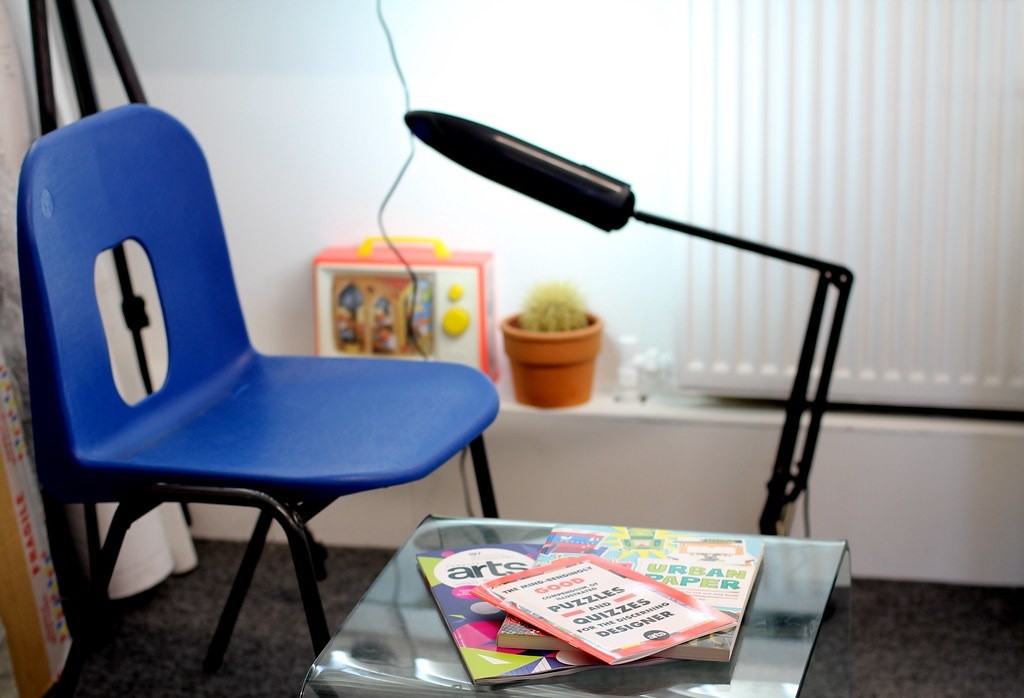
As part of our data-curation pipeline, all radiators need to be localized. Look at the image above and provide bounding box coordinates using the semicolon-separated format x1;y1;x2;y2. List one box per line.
676;0;1023;410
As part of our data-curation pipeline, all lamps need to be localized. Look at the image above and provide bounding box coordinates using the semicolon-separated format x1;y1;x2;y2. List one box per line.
404;111;855;627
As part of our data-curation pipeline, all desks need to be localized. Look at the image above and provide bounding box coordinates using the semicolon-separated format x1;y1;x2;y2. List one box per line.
301;512;855;698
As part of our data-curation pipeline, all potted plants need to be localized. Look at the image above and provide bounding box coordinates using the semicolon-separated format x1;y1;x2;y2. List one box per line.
500;281;603;408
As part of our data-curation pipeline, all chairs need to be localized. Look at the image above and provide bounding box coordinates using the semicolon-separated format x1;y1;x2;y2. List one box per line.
17;106;499;697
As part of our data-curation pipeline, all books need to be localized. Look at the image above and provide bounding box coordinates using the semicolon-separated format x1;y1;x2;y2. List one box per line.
417;522;766;684
